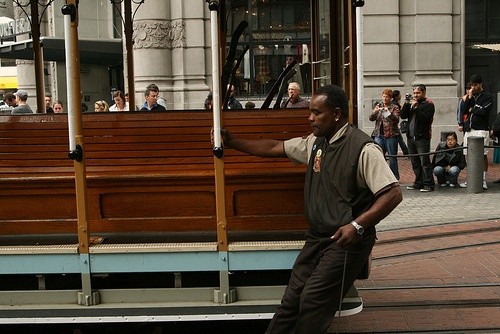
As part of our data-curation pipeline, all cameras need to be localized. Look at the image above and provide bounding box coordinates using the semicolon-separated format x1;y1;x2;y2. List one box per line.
404;94;412;100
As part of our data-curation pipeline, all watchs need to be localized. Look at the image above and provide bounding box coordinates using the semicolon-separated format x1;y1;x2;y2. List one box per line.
351;221;364;235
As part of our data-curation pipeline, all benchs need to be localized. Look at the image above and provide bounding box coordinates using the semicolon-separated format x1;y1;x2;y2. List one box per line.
0;107;310;236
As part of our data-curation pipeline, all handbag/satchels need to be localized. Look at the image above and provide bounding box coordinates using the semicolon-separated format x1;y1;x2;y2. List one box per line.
400;120;407;134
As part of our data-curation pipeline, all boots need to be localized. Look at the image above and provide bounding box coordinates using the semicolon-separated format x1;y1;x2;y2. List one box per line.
459;181;468;188
483;171;488;189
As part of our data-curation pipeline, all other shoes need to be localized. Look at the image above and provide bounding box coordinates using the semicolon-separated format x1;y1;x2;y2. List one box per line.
420;186;434;192
407;185;420;190
441;183;448;187
449;183;456;188
405;156;411;160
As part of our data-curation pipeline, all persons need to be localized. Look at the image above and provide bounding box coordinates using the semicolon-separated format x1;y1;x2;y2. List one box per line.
141;85;167;110
457;75;500;189
211;85;402;334
392;90;410;158
369;90;400;186
400;84;435;191
432;132;466;186
36;96;54;113
53;101;64;113
204;84;255;109
109;91;139;111
95;100;109;112
282;82;309;107
3;90;33;114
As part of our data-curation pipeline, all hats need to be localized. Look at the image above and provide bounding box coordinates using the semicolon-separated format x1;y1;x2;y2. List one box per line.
13;89;28;98
471;73;482;83
384;89;392;96
464;82;471;89
